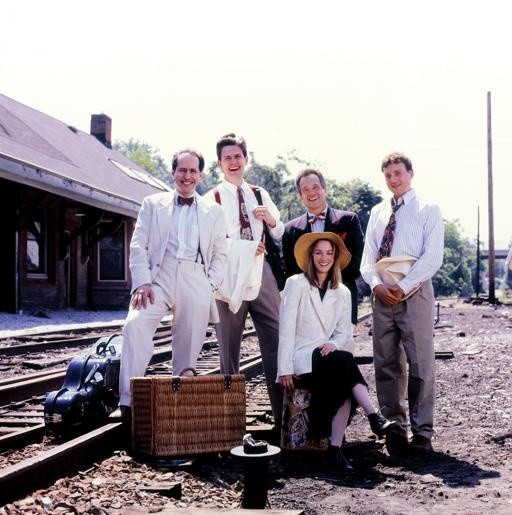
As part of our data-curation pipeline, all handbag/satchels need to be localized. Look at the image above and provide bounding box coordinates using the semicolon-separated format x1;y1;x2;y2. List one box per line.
252;185;286;291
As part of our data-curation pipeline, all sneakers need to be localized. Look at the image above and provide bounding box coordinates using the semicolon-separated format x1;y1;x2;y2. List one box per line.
387;428;408;451
408;435;433;453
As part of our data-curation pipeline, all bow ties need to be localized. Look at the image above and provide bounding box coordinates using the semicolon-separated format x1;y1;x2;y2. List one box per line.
308;212;326;225
177;196;194;206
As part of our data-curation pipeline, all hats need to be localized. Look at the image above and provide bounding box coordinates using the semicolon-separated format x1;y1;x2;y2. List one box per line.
293;231;353;273
374;256;425;301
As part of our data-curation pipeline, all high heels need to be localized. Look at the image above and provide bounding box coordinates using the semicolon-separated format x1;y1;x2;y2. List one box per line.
329;443;355;473
367;412;397;441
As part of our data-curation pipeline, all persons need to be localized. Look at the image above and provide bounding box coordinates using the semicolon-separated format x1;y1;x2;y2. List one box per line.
358;154;444;455
107;149;231;421
281;166;365;325
274;230;399;479
201;134;283;443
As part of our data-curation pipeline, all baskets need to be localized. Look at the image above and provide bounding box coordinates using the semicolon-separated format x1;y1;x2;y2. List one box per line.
128;366;250;459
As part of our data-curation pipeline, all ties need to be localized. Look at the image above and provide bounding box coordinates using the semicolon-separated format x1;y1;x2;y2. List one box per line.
236;186;254;240
376;199;404;262
